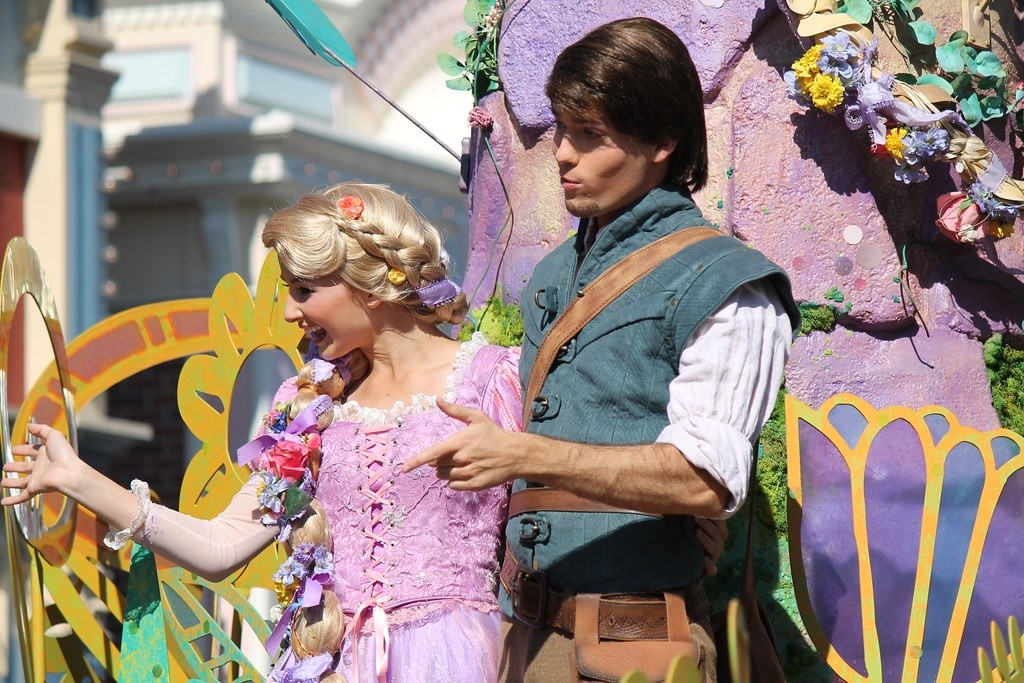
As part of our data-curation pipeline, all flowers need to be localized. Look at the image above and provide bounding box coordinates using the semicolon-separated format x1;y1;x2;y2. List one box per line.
340;196;365;221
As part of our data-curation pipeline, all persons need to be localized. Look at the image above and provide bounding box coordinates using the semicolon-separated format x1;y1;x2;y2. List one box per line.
404;16;803;682
0;184;731;683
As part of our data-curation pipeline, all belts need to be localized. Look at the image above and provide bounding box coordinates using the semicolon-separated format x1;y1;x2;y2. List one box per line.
499;539;709;640
